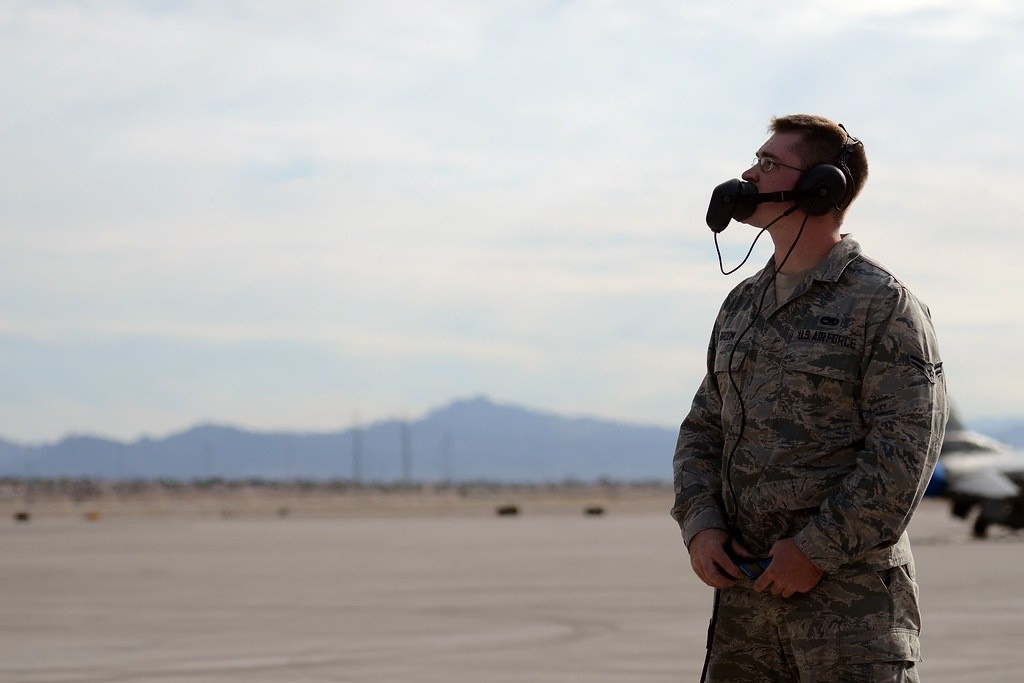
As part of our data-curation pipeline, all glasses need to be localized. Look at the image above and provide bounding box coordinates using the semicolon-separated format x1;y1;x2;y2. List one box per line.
751;154;802;174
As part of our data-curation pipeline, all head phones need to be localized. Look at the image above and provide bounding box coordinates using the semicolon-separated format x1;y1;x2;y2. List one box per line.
792;134;860;218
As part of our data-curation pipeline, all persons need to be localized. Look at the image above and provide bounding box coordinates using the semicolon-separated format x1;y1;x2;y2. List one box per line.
671;115;951;683
